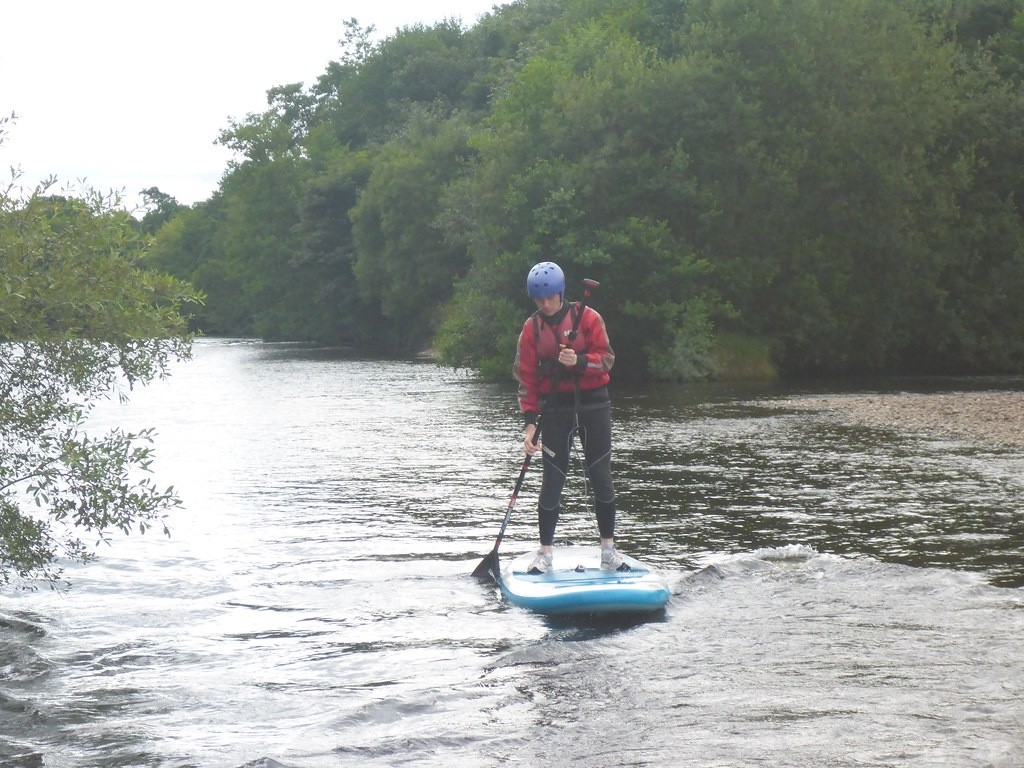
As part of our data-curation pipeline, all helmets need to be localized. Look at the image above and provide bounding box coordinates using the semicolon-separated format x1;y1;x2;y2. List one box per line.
526;262;565;302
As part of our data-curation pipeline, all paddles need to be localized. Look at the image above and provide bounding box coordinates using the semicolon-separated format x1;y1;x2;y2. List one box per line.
473;276;601;577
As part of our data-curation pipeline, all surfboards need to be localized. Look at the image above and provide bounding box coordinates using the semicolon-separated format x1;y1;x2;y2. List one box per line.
497;543;669;614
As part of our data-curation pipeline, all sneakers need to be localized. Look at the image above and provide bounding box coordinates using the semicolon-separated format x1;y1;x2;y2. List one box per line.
601;548;630;572
527;551;553;573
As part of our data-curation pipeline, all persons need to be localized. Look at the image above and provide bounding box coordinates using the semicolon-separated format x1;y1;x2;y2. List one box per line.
513;262;633;575
